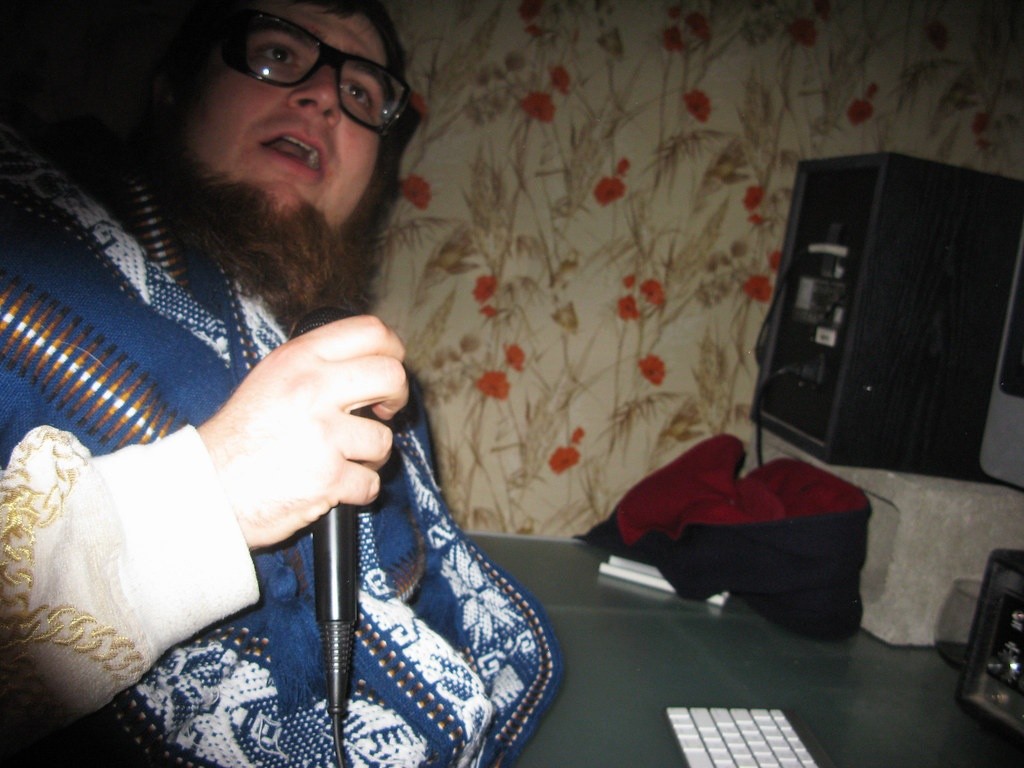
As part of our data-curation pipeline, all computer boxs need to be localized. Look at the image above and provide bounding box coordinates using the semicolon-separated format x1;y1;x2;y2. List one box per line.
751;152;1024;485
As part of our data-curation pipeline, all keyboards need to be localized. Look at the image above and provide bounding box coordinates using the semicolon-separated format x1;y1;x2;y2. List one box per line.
666;705;836;768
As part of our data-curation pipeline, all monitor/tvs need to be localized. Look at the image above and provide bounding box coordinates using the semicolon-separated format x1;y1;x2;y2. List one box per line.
979;227;1024;491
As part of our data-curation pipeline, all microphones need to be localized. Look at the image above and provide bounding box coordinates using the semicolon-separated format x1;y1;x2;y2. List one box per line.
286;305;358;717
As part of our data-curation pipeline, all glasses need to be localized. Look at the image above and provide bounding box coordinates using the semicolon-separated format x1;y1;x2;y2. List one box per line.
222;8;414;139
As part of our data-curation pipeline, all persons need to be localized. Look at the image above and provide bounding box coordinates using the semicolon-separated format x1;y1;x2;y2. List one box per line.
1;0;566;768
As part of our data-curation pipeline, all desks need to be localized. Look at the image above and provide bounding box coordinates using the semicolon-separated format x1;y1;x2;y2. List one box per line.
460;535;1024;768
